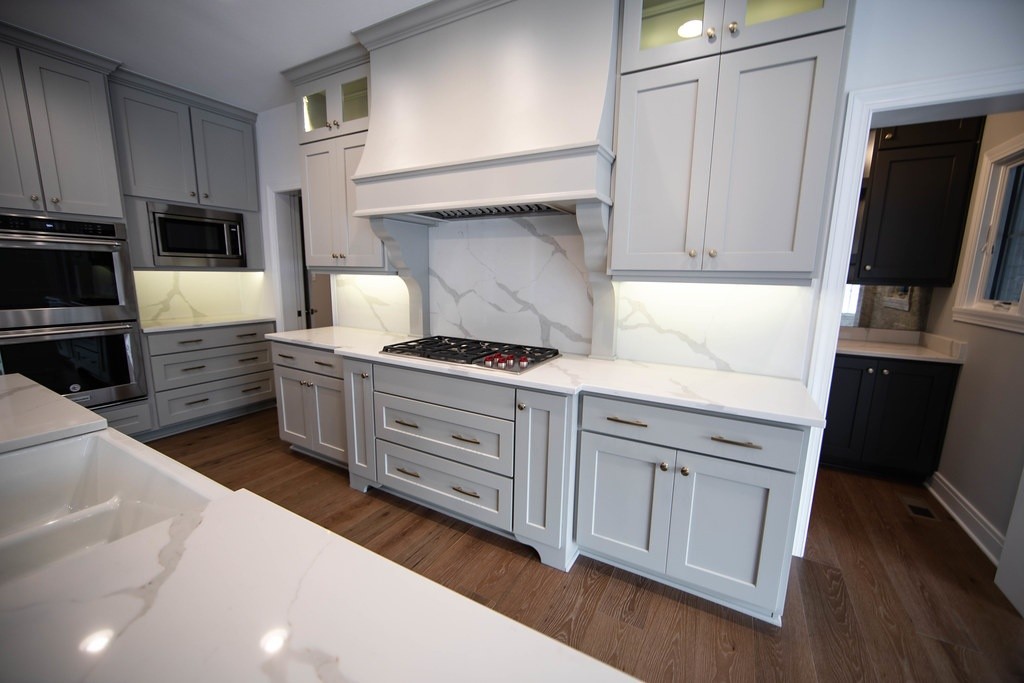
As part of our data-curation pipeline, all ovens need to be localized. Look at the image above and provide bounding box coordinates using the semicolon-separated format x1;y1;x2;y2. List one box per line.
1;207;149;411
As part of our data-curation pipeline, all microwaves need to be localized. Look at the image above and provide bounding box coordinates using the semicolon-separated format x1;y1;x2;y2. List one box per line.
146;201;247;267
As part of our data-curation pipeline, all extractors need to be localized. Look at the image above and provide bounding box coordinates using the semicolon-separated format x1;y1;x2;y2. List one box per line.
351;0;632;226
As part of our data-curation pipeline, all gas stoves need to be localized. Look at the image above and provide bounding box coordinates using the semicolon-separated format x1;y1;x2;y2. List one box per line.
379;335;563;374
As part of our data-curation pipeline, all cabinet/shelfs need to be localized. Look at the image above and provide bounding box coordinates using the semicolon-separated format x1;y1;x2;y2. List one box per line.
143;322;276;437
0;21;127;224
846;116;988;290
294;62;371;142
299;133;397;277
605;29;854;287
820;355;962;489
342;356;579;572
574;392;811;628
270;341;348;469
109;67;260;213
